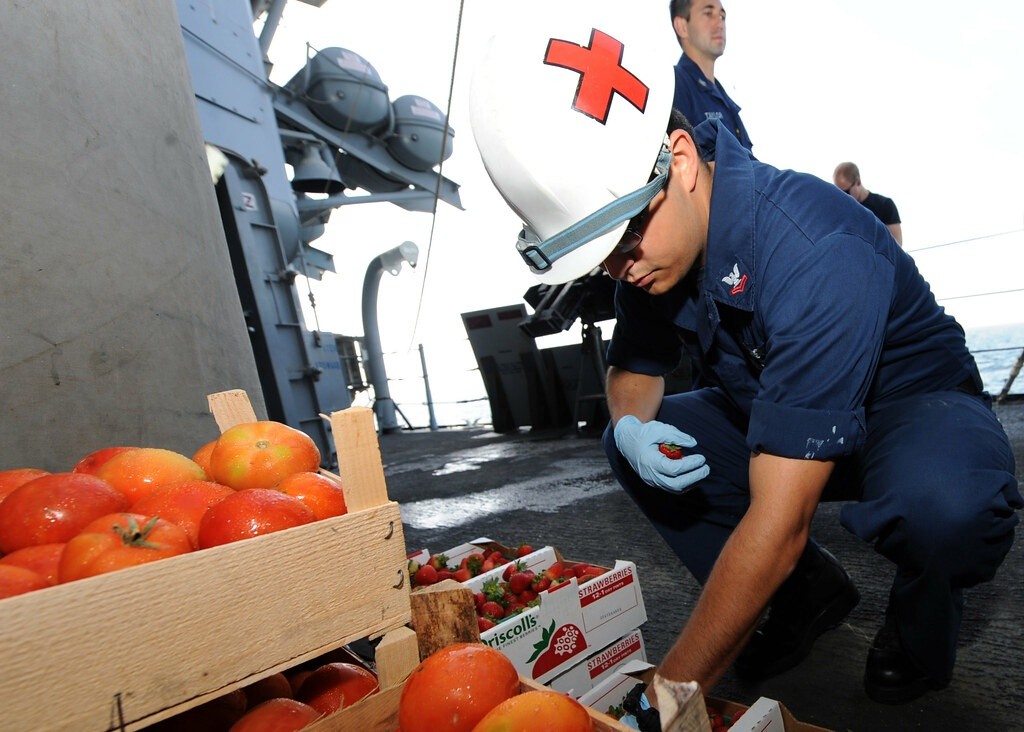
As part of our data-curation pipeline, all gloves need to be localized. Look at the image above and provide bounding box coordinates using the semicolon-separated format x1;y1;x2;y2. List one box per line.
613;415;710;494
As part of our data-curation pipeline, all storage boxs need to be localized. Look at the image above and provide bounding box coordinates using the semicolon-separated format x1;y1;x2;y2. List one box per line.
0;388;834;732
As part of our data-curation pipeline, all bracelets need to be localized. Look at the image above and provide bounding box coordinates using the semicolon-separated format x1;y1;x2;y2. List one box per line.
623;681;663;731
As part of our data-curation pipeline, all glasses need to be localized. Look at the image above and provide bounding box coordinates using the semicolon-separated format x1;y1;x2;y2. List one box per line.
843;179;856;196
612;205;649;255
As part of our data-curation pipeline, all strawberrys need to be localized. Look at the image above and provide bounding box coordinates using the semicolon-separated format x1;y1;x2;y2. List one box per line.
605;698;748;732
407;544;534;593
468;560;611;632
659;443;682;460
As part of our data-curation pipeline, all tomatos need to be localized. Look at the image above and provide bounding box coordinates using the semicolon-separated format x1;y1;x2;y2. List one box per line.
0;421;348;602
135;642;594;732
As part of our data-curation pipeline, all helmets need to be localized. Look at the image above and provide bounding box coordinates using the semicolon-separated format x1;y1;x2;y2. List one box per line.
473;19;676;285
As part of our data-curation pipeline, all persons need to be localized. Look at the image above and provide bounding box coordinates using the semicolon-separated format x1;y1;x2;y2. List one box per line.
828;153;906;255
593;99;1022;731
669;0;758;160
